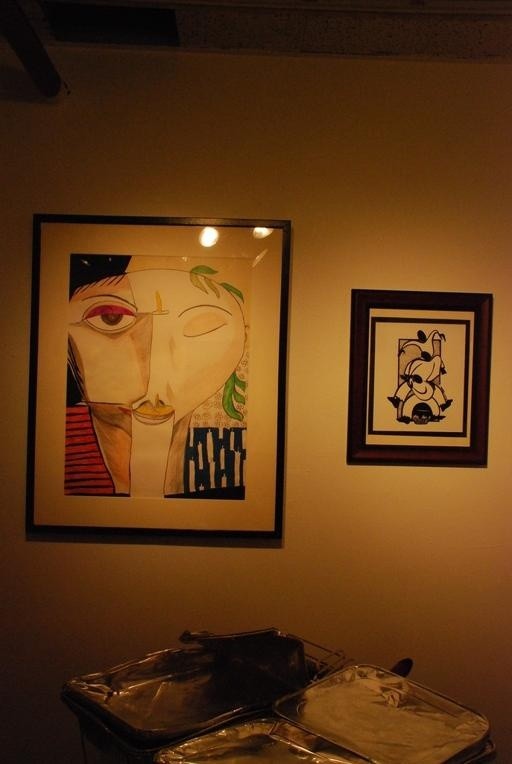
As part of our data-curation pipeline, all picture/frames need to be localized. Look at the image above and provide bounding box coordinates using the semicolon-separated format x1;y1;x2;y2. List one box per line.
346;288;495;469
25;210;290;550
